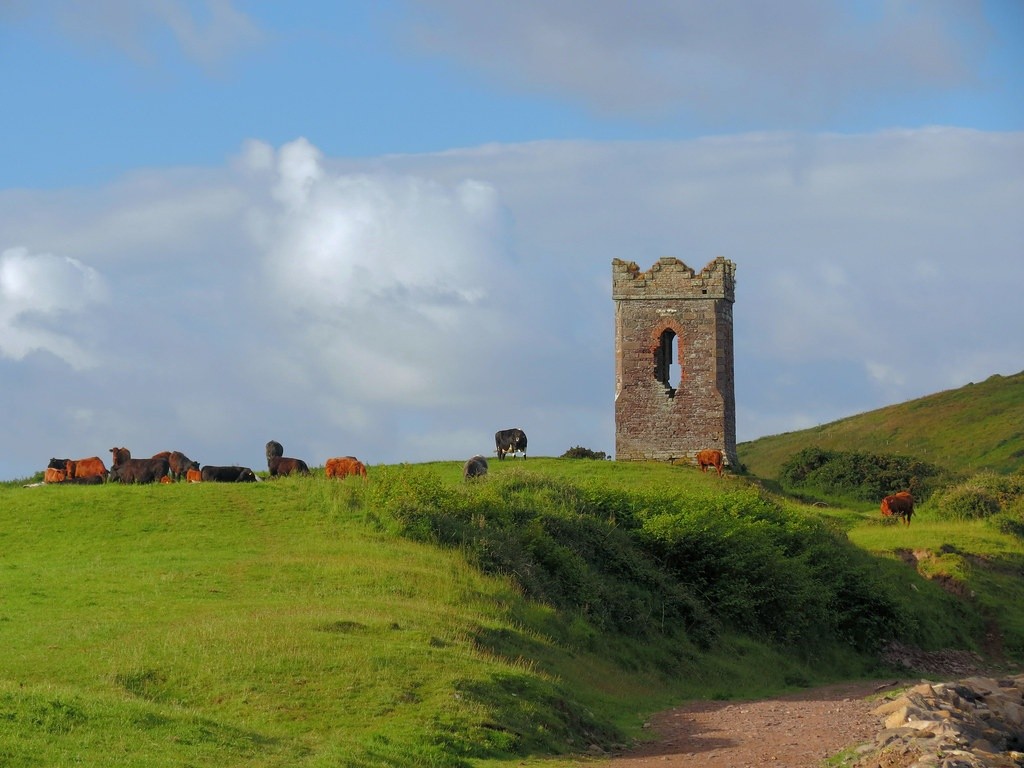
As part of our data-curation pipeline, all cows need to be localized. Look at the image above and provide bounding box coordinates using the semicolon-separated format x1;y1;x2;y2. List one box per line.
462;454;488;483
695;449;725;476
44;435;367;488
494;428;528;461
880;491;917;526
